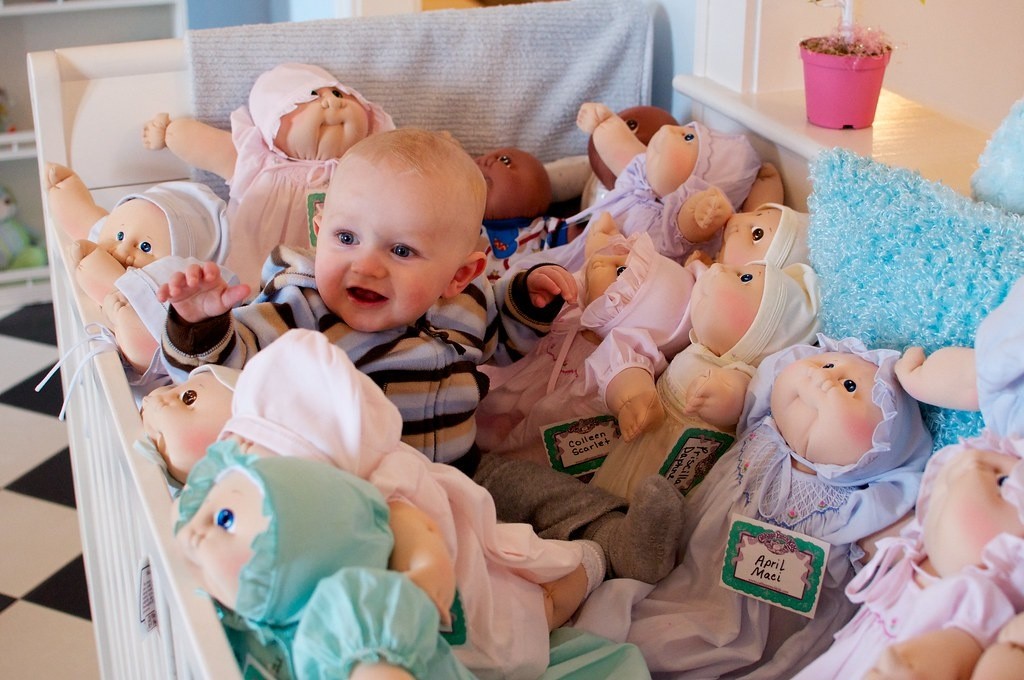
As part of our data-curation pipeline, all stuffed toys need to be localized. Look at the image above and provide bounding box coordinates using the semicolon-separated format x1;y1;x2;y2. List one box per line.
0;185;49;287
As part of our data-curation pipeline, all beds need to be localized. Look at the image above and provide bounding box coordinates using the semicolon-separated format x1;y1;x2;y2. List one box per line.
22;0;660;679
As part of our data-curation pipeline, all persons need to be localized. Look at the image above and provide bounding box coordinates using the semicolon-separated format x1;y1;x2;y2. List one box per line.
46;64;1024;680
158;127;688;583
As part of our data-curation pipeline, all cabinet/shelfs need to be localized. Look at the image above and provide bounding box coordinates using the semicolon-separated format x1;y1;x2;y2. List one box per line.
1;1;189;286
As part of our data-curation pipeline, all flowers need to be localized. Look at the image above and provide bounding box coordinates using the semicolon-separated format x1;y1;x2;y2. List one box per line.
820;23;889;60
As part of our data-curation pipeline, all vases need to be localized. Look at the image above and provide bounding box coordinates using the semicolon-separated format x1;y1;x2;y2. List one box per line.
798;36;895;130
